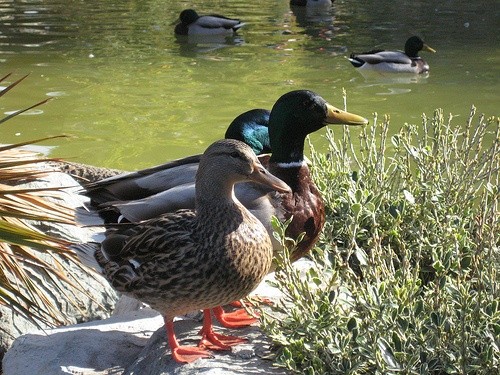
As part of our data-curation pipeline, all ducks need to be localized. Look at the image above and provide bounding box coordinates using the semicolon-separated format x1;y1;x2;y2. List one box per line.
344;35;436;74
73;89;370;364
168;8;248;37
